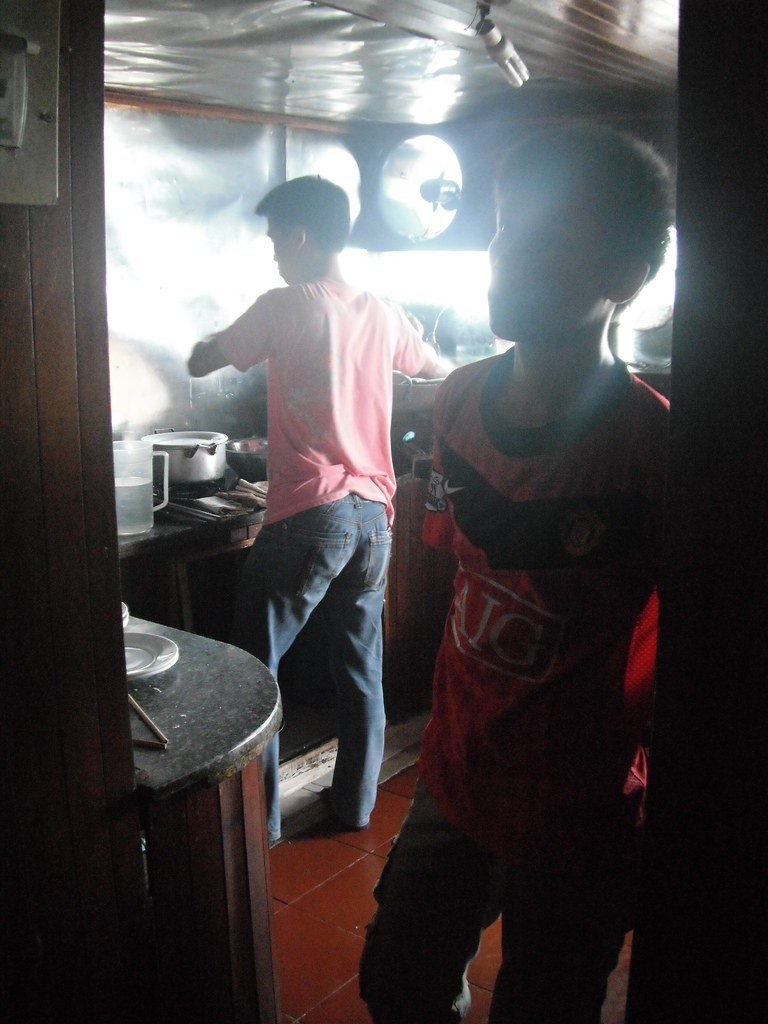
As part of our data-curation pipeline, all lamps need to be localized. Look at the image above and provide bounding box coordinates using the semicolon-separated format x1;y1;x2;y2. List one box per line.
475;4;531;89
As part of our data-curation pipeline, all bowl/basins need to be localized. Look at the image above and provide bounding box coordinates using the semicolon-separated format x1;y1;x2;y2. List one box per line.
225;436;269;482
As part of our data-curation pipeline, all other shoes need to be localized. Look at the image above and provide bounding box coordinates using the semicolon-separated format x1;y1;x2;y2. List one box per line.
321;786;370;832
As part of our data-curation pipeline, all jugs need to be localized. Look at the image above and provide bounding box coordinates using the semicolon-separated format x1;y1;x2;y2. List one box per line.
111;441;170;536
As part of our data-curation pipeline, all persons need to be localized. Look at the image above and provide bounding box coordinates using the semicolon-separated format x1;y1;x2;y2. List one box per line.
186;170;441;850
352;125;672;1024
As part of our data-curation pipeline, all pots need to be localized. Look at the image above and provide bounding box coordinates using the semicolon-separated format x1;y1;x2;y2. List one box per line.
140;428;229;485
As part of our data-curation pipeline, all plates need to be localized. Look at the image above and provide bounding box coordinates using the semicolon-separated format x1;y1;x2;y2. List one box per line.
123;632;179;682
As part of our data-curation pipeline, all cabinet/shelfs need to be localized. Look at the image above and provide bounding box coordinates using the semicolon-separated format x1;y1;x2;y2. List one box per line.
114;447;457;1024
